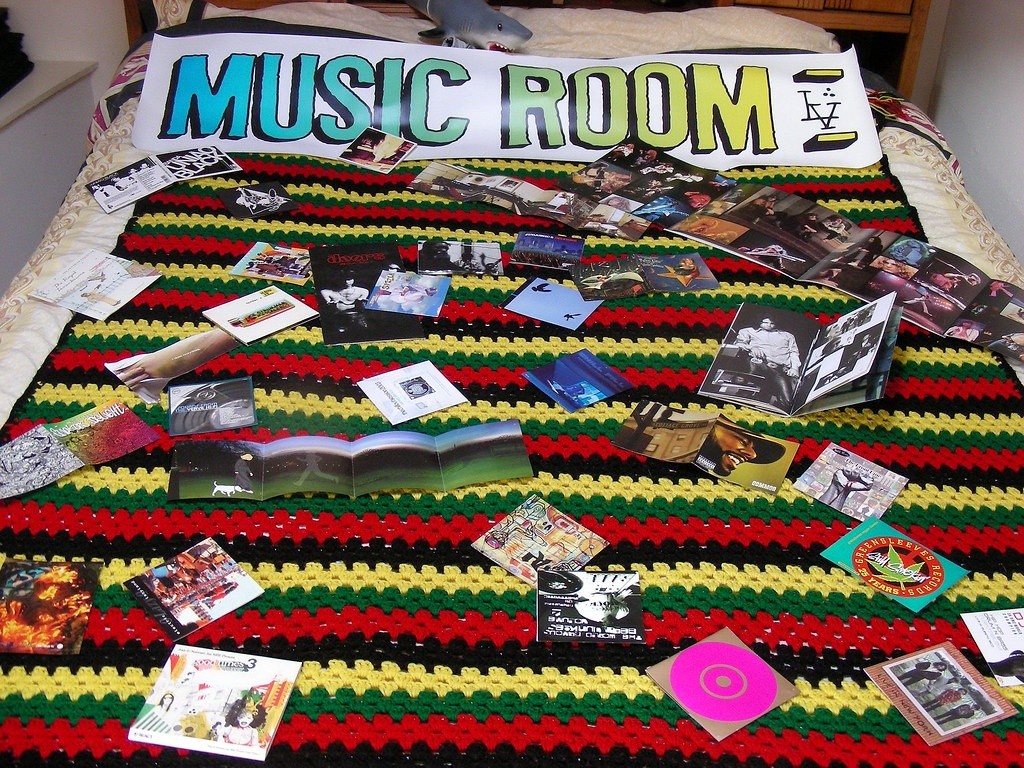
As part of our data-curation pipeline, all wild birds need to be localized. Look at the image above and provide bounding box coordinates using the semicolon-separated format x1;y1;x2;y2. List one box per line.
531;282;553;293
563;313;581;322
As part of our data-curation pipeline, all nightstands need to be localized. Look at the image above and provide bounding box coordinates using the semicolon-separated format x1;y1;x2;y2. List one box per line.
0;59;98;301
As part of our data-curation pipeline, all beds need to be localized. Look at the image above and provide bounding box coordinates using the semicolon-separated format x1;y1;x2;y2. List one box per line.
0;0;1024;768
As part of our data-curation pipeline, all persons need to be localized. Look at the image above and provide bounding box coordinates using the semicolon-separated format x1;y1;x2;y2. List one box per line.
567;141;1023;727
106;326;240;403
320;267;370;328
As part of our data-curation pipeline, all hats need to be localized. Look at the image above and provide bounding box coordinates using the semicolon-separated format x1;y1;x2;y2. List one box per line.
717;415;786;464
241;454;253;461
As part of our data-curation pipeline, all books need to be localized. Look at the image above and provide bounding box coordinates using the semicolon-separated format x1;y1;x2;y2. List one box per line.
407;159;1024;748
552;136;737;228
943;276;1024;362
0;126;535;768
664;180;991;338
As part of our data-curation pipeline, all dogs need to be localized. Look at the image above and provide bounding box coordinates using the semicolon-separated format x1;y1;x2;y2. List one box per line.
211;480;241;497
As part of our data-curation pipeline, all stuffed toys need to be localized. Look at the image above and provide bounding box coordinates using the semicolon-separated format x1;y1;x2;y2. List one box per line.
406;0;533;52
